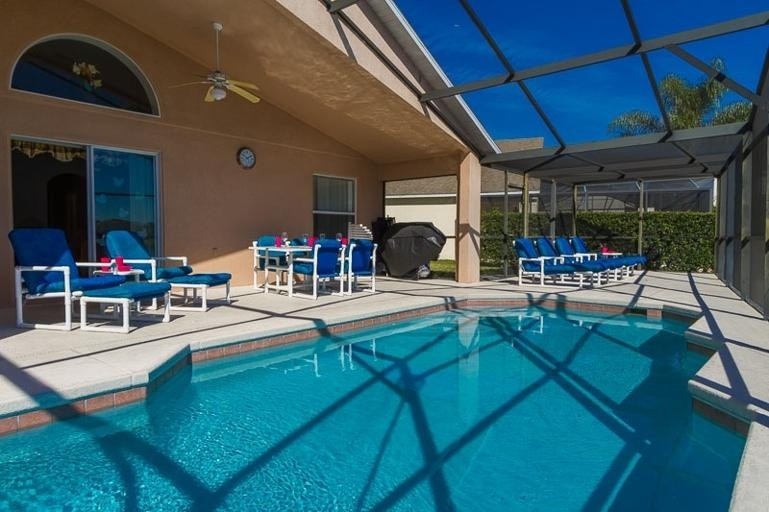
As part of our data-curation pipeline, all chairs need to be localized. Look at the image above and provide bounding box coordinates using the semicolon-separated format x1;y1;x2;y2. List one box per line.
8;227;127;334
512;237;603;290
103;229;193;312
267;345;345;377
247;234;379;301
517;314;543;334
345;338;378;370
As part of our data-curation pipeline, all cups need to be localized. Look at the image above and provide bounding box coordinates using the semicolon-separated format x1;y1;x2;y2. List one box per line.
281;232;342;243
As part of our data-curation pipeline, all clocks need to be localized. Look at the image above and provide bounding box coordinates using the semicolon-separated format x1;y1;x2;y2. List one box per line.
236;147;256;170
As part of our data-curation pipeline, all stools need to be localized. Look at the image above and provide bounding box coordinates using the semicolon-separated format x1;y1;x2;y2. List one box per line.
168;273;232;312
545;256;648;290
80;281;172;333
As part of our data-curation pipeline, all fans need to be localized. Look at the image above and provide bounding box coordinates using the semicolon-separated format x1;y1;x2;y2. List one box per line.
167;22;261;105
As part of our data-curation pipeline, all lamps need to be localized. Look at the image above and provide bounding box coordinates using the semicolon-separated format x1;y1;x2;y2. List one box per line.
211;86;227;101
